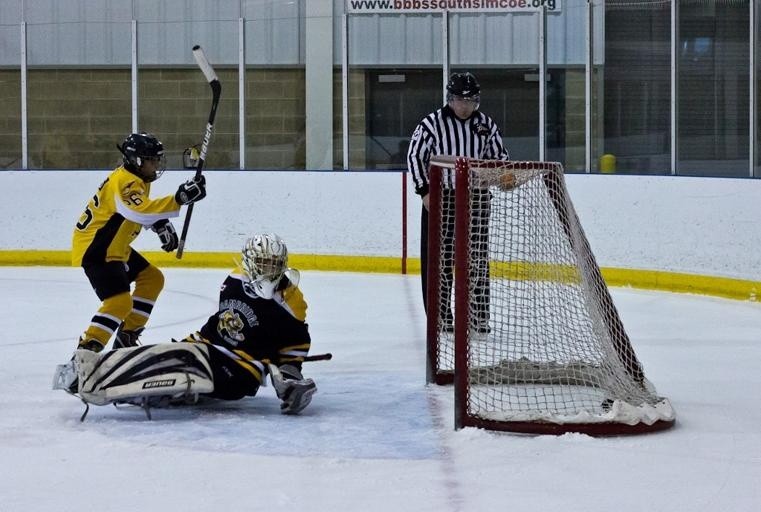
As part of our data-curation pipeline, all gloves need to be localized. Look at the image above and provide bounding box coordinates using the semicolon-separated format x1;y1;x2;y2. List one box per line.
152;218;178;253
275;379;318;415
176;176;207;205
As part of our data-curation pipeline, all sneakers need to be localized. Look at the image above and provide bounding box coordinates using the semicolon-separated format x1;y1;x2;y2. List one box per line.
470;320;491;334
440;322;454;334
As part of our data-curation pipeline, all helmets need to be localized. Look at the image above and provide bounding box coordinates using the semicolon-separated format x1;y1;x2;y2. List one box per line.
446;73;481;111
116;132;168;182
240;234;288;301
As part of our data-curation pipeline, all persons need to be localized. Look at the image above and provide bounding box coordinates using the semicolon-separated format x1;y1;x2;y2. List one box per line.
72;131;206;356
75;232;317;417
407;73;517;336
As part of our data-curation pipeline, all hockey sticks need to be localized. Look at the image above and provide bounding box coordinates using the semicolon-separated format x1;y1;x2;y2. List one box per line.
176;42;222;259
